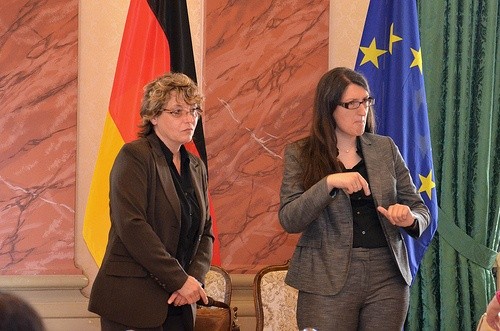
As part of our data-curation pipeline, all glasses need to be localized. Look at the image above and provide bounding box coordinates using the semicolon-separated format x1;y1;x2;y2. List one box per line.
157;107;203;117
334;97;376;110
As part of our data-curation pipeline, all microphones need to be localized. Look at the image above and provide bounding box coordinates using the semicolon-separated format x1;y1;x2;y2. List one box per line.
195;296;229;309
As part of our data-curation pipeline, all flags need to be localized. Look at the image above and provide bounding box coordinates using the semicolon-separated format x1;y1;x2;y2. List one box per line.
82;0;222;269
353;0;439;286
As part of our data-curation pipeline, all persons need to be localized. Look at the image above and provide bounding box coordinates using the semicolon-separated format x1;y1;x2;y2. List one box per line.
88;72;216;331
277;68;432;331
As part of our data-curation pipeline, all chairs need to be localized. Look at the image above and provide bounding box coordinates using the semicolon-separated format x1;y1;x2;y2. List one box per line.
252;259;300;331
195;265;240;331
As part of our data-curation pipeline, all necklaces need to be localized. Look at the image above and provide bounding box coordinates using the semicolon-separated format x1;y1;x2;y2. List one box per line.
336;135;355;153
